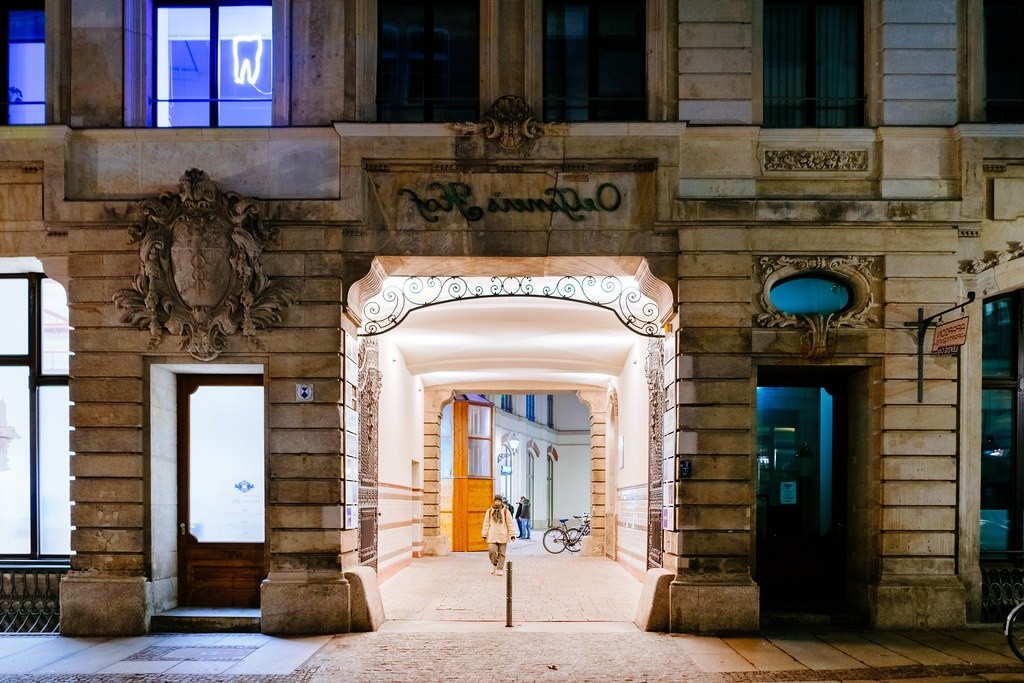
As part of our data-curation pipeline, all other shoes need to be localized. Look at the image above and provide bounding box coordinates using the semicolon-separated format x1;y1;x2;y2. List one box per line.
490;563;497;574
517;535;522;538
497;569;503;576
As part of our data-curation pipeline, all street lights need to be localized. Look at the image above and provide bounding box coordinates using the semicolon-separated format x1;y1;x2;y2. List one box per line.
503;429;520;517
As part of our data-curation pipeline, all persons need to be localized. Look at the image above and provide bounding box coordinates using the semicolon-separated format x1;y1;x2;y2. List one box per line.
503;496;531;538
482;494;516;576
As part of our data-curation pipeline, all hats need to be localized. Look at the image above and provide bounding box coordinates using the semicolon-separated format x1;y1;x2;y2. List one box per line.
494;496;503;503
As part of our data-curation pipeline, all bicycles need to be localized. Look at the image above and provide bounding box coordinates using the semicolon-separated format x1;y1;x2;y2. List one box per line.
542;512;592;554
1002;599;1024;663
